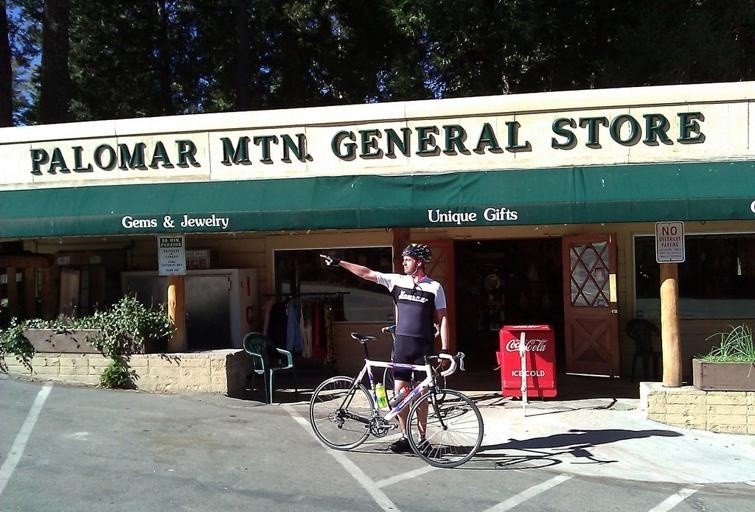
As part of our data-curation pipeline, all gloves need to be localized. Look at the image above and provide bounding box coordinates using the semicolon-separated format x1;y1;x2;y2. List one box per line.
437;350;449;370
319;254;340;267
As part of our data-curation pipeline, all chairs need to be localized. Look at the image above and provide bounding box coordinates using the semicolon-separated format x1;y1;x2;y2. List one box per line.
243;331;298;404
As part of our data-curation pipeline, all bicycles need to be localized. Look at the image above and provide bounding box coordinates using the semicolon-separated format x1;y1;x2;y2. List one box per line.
309;322;483;469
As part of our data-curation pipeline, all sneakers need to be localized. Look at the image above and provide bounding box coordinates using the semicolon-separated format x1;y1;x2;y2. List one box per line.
388;437;411;452
418;439;435;455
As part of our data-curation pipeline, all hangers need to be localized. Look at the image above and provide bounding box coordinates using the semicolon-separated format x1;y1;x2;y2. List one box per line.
262;295;334;310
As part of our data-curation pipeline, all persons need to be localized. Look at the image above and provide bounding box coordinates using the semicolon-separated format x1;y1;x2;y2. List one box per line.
320;243;448;457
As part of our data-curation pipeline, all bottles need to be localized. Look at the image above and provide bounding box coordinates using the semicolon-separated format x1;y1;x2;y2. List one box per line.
374;381;388;408
388;386;412;407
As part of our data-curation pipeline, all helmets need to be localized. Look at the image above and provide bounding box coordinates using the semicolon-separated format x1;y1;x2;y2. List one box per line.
402;243;433;263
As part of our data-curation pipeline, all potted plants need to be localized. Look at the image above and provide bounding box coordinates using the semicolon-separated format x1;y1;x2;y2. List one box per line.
692;324;754;391
